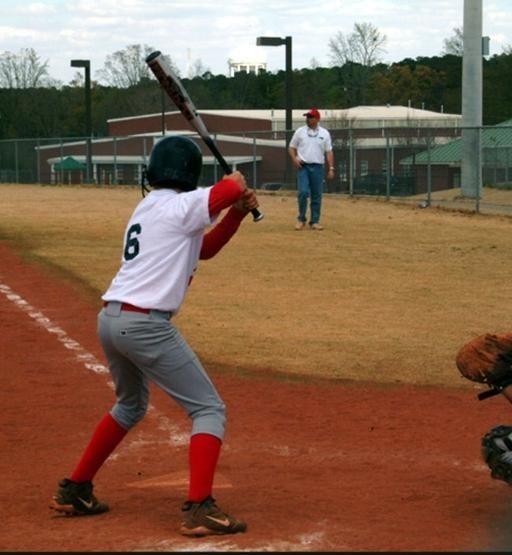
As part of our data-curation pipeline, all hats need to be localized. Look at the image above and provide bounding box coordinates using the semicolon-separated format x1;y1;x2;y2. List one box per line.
303;109;320;120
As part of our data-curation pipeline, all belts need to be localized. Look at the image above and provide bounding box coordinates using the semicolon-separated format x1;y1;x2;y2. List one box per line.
103;300;175;321
299;162;321;166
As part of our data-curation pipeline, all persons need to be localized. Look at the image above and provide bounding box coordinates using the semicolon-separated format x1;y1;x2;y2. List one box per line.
288;109;334;230
49;135;260;537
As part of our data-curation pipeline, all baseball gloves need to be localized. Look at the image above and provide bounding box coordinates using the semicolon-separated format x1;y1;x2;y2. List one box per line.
456;332;511;399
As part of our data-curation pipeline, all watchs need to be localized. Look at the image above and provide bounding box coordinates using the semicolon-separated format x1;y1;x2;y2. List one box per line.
329;167;335;170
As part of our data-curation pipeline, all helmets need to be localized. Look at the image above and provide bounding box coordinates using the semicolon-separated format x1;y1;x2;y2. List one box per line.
148;137;203;193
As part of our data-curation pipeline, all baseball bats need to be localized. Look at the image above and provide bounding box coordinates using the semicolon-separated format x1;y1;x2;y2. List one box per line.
146;50;263;222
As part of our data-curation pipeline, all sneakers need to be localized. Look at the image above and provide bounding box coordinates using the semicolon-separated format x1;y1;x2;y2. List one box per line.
180;500;246;536
311;223;324;229
296;220;305;230
48;477;108;512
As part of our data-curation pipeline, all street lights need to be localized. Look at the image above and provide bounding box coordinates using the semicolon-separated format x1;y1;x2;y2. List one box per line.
256;37;292;183
71;60;92;183
